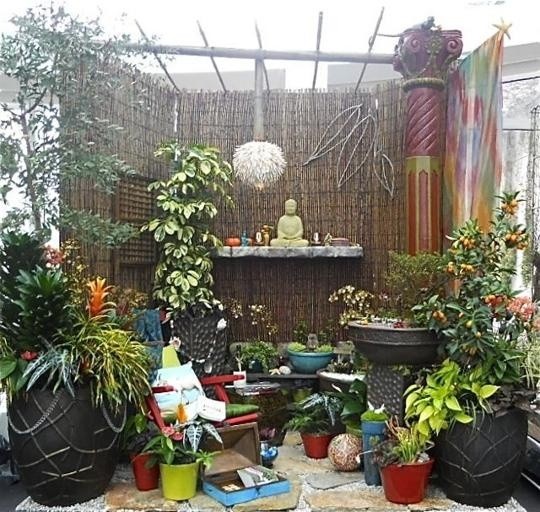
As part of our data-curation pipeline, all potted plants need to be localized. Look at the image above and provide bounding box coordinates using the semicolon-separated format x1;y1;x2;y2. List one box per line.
154;425;204;501
344;379;367;445
330;281;441;365
370;419;437;500
419;189;536;507
232;297;283;373
151;146;231;366
284;318;334;373
291;392;342;460
129;427;164;491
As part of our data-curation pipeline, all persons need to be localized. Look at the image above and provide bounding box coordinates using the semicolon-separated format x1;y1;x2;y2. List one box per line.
269;198;309;247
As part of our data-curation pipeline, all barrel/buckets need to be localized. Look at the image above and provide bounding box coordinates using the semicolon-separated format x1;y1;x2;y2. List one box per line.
373;439;436;506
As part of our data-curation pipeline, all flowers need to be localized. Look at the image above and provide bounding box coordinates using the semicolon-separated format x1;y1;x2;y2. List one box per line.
23;246;121;366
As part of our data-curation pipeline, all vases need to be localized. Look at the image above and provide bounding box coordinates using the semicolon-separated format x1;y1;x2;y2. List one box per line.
0;364;131;505
